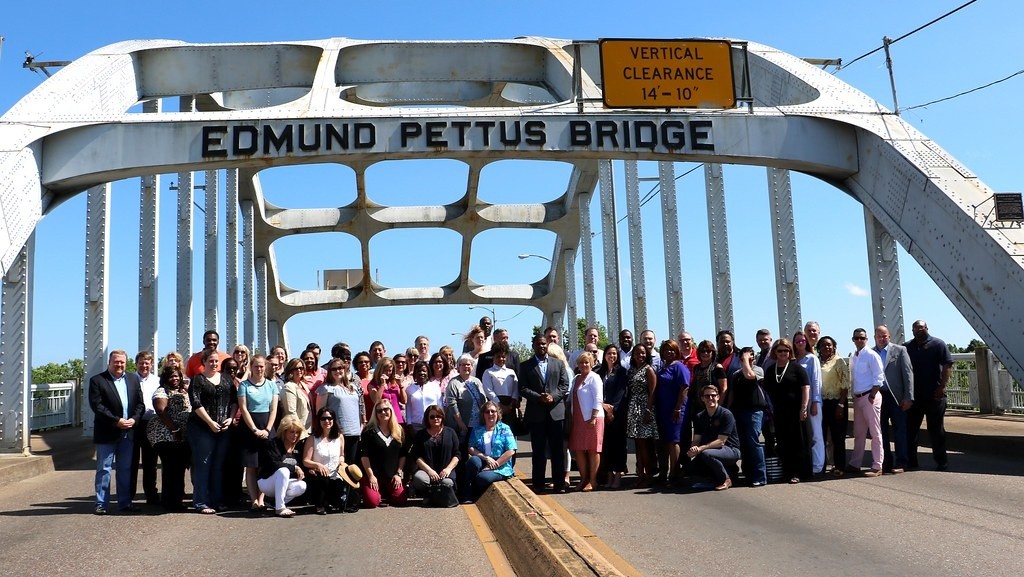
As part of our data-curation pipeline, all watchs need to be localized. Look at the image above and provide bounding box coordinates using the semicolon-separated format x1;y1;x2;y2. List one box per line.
397;472;402;477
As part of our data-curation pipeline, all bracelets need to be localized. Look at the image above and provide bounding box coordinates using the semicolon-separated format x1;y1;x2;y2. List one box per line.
838;403;845;408
800;409;807;413
674;409;681;412
399;386;404;389
252;428;258;432
171;427;181;432
870;395;875;399
266;428;270;434
362;421;368;423
645;408;651;413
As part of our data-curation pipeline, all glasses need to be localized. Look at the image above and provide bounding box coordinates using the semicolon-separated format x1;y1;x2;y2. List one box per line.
853;336;866;341
320;417;333;422
775;348;791;353
407;353;419;359
357;360;371;365
484;410;498;414
428;414;443;420
293;366;305;371
793;338;806;346
340;356;351;361
331;366;345;371
703;394;719;399
700;349;712;354
585;350;598;353
394;360;407;366
234;350;246;355
225;366;238;371
459;363;472;368
376;408;391;414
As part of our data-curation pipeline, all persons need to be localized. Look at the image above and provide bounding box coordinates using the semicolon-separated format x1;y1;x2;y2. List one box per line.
88;351;146;514
147;316;850;518
870;325;915;473
846;329;891;478
131;350;160;506
901;320;955;471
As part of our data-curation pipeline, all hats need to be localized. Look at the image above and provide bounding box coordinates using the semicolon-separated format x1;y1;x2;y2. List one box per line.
337;463;364;489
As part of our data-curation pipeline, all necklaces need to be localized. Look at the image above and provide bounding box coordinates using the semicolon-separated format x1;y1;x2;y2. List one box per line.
775;360;790;383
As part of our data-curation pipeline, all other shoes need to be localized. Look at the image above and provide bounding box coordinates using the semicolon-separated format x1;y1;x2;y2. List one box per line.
92;486;482;516
531;445;954;492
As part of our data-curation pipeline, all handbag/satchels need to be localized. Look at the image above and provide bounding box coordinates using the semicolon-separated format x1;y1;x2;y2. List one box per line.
420;481;459;508
509;408;529;436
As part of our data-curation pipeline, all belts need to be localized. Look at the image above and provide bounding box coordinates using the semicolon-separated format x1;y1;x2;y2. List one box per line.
854;389;873;398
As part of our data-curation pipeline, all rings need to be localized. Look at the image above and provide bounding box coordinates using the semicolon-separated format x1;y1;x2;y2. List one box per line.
840;413;842;415
